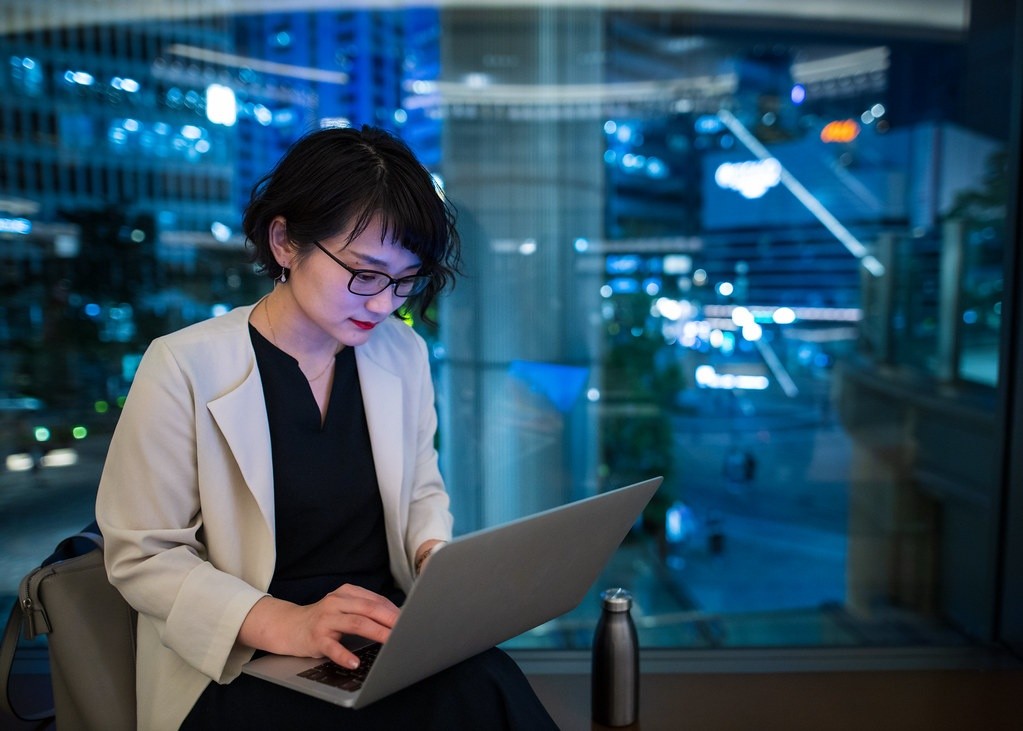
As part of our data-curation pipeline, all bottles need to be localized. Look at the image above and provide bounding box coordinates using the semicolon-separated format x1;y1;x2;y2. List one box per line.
591;588;639;728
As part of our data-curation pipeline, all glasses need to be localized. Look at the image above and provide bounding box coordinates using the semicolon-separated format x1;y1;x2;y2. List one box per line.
310;236;435;298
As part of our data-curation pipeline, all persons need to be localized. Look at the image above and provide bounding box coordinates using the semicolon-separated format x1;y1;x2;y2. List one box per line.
94;123;561;730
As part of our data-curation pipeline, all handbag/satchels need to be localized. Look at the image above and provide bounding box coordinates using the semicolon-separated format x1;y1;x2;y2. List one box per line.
1;528;138;731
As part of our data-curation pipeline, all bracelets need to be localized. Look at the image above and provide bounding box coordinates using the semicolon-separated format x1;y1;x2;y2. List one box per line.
417;547;432;571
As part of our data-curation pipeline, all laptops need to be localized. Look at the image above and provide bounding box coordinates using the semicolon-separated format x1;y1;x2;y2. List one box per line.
240;476;665;707
